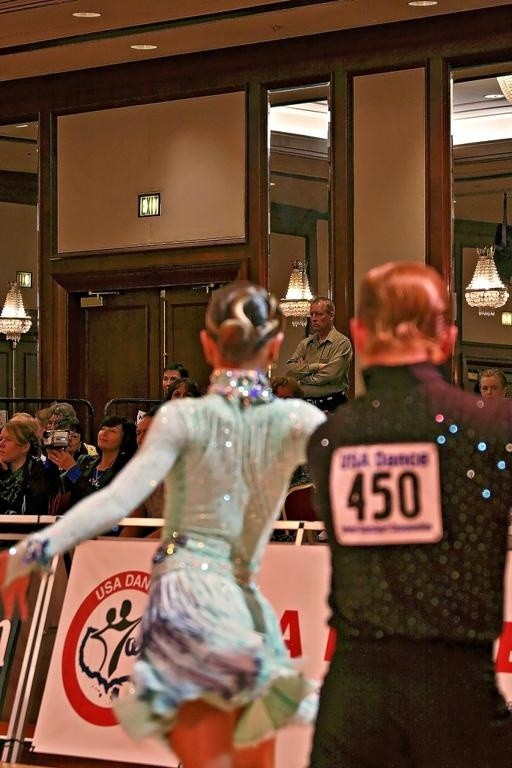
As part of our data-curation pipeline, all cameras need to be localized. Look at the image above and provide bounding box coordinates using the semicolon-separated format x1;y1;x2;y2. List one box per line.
43;430;69;447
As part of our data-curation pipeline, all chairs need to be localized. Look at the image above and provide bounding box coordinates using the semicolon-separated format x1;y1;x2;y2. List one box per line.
280;483;323;541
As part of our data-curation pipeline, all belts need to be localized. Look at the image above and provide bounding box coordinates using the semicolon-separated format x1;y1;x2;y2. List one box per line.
307;392;345;405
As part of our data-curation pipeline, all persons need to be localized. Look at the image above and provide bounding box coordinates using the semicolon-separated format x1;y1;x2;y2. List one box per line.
3;295;512;542
1;278;327;768
306;261;512;767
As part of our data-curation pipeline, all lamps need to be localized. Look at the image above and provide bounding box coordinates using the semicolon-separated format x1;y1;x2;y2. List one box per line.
278;254;317;329
463;238;509;318
2;271;32;342
